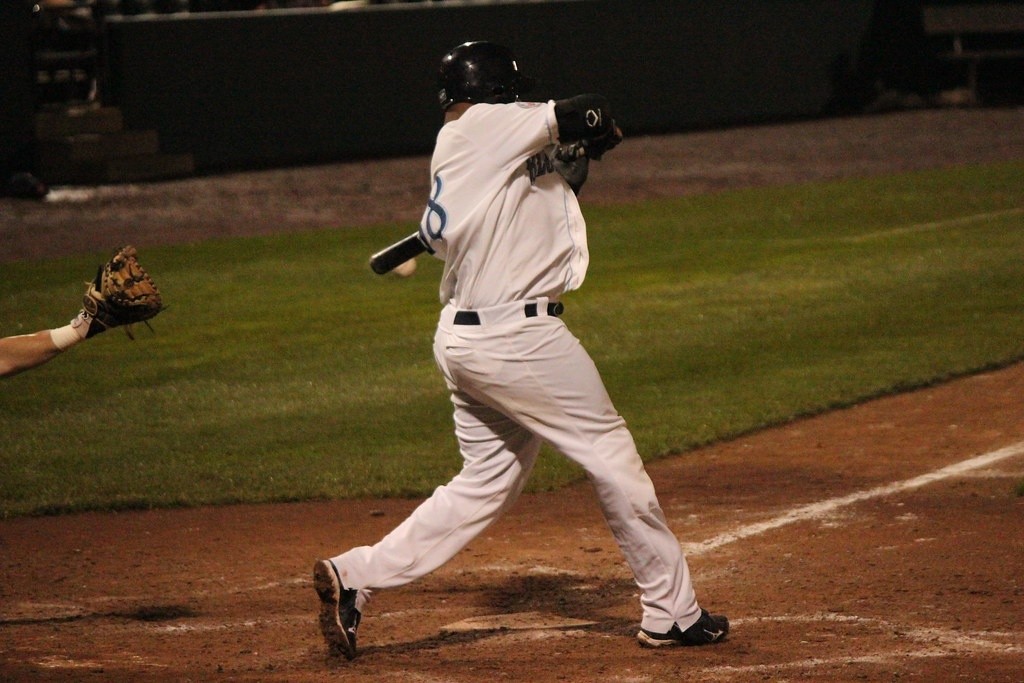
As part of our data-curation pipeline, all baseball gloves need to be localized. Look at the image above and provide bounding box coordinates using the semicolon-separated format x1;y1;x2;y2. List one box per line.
81;244;169;342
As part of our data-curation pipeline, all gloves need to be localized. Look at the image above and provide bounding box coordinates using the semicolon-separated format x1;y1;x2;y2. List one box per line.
582;119;623;161
548;143;589;197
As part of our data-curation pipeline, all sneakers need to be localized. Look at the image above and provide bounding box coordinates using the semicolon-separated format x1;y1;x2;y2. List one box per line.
314;558;360;661
638;608;730;647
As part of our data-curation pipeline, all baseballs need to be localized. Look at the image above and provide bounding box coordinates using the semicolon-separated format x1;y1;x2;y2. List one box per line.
394;259;417;277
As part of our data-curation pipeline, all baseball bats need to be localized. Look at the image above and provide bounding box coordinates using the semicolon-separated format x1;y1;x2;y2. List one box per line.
368;126;625;275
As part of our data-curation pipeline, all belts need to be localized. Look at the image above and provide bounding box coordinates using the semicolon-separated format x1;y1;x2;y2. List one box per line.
454;302;564;325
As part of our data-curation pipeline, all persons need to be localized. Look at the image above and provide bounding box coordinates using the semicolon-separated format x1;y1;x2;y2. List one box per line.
0;244;161;379
311;40;729;657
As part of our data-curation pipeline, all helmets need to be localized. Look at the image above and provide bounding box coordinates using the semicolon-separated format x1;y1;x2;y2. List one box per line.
437;40;523;109
